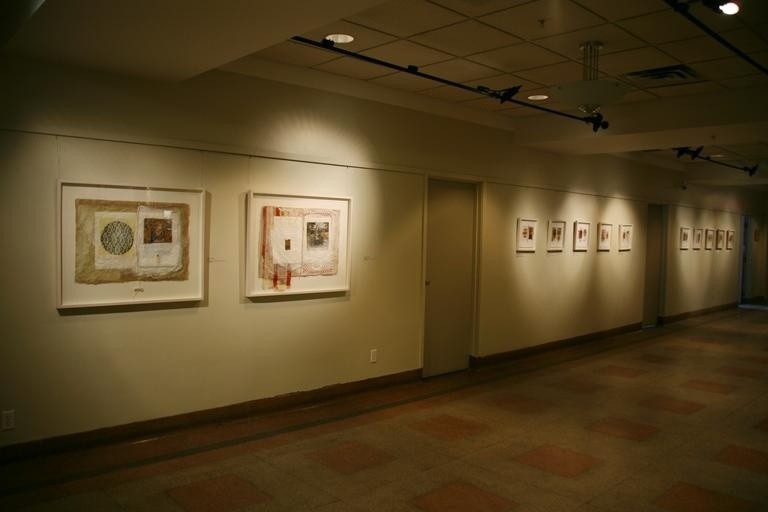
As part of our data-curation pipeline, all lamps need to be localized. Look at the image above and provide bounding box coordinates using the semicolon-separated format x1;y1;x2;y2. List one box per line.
744;164;759;177
498;85;521;105
590;111;603;132
689;146;704;160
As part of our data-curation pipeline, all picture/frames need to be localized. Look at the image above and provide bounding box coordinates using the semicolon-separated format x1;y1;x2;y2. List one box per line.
244;189;353;299
515;216;633;254
55;179;209;311
679;227;735;251
